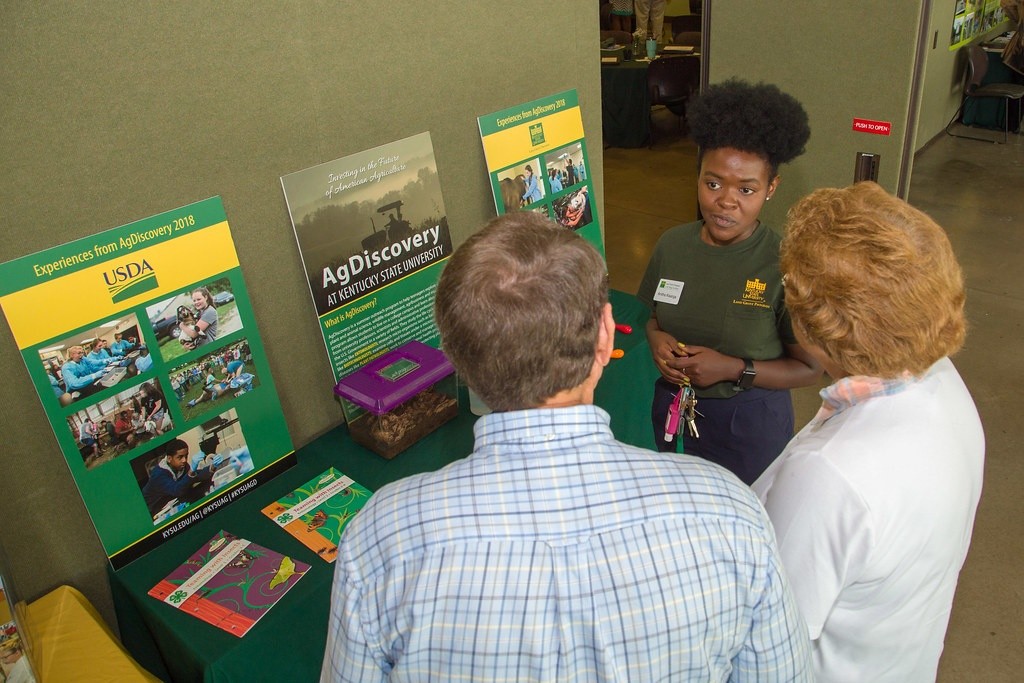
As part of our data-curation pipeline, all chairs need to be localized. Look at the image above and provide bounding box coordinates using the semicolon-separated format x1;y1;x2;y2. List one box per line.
943;45;1024;144
643;56;700;149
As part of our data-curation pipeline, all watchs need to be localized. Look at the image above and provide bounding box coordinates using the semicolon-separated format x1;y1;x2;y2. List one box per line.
737;358;756;391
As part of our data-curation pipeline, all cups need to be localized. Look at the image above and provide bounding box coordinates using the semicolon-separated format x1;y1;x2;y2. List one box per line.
623;49;632;61
646;39;657;59
468;387;494;416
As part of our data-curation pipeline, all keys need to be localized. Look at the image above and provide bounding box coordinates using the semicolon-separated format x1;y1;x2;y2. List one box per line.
677;397;706;438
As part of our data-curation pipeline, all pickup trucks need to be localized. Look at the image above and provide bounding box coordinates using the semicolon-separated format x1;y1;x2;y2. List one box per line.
152;313;181;340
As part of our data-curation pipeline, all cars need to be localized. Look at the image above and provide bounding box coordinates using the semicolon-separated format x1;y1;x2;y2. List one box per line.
212;291;234;307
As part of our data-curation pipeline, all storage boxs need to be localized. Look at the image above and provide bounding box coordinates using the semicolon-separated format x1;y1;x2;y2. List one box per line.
334;339;457;460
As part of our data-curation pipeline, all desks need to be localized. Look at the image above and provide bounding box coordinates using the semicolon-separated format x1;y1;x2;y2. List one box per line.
601;46;651;148
107;290;683;683
27;584;164;683
961;33;1024;132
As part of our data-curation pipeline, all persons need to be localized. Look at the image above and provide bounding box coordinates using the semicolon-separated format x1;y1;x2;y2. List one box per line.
142;439;216;516
0;646;36;683
609;0;633;32
549;159;585;195
636;77;824;487
553;186;593;231
171;342;253;406
80;377;173;459
499;165;542;213
634;0;670;45
45;333;154;407
183;288;218;352
749;180;987;682
318;210;815;683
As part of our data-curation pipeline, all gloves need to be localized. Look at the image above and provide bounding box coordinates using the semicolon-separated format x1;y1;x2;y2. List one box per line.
213;454;223;467
191;452;206;472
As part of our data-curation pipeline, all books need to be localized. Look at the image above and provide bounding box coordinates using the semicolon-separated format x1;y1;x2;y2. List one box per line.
0;576;36;683
261;467;374;563
147;530;313;639
